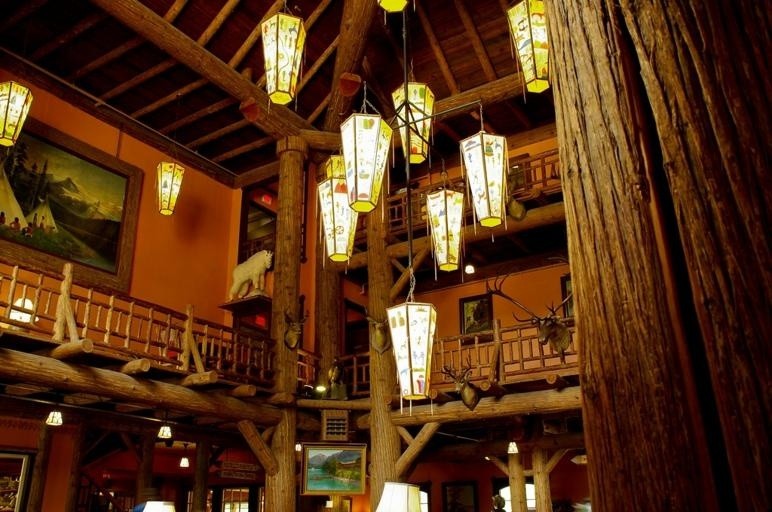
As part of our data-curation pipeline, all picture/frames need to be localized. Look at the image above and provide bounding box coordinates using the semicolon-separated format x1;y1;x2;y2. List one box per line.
561;273;575;327
300;445;367;496
0;112;145;297
459;293;493;344
1;453;32;511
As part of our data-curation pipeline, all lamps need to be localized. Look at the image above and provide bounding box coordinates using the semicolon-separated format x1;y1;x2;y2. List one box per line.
157;405;172;439
261;0;308;106
506;0;551;93
179;440;191;467
0;82;34;147
387;266;437;417
45;393;64;428
393;57;435;164
317;174;360;270
377;0;407;14
296;426;304;453
340;82;394;214
326;151;346;180
427;155;464;272
157;142;185;216
462;100;512;231
506;415;520;455
